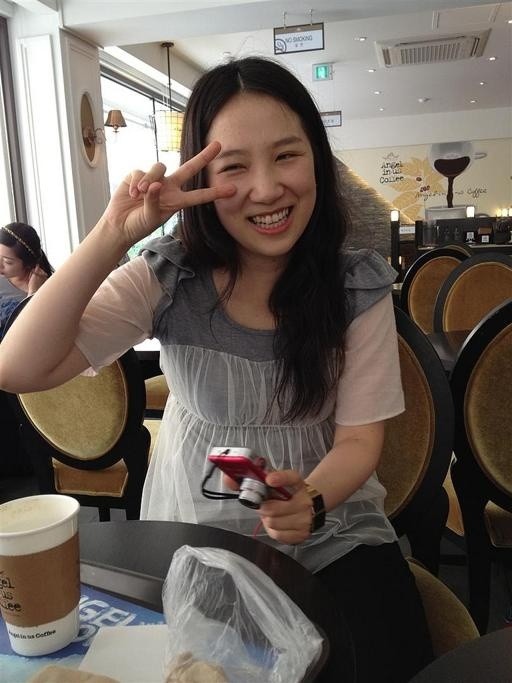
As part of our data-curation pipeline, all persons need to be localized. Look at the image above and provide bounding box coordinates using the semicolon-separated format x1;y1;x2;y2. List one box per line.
0;220;56;331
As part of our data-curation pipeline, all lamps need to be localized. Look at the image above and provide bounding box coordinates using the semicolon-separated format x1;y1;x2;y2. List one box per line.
154;42;186;153
83;110;127;147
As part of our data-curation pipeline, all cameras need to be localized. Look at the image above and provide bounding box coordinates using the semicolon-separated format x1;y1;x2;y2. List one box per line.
205;446;293;510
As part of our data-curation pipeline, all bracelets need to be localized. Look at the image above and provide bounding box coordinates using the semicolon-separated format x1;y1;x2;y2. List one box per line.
0;53;437;683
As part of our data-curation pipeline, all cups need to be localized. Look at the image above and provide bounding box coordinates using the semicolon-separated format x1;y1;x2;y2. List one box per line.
0;494;84;660
424;203;489;221
427;141;487;178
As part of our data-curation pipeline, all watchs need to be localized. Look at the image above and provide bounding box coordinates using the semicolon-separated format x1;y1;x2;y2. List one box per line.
304;483;327;532
